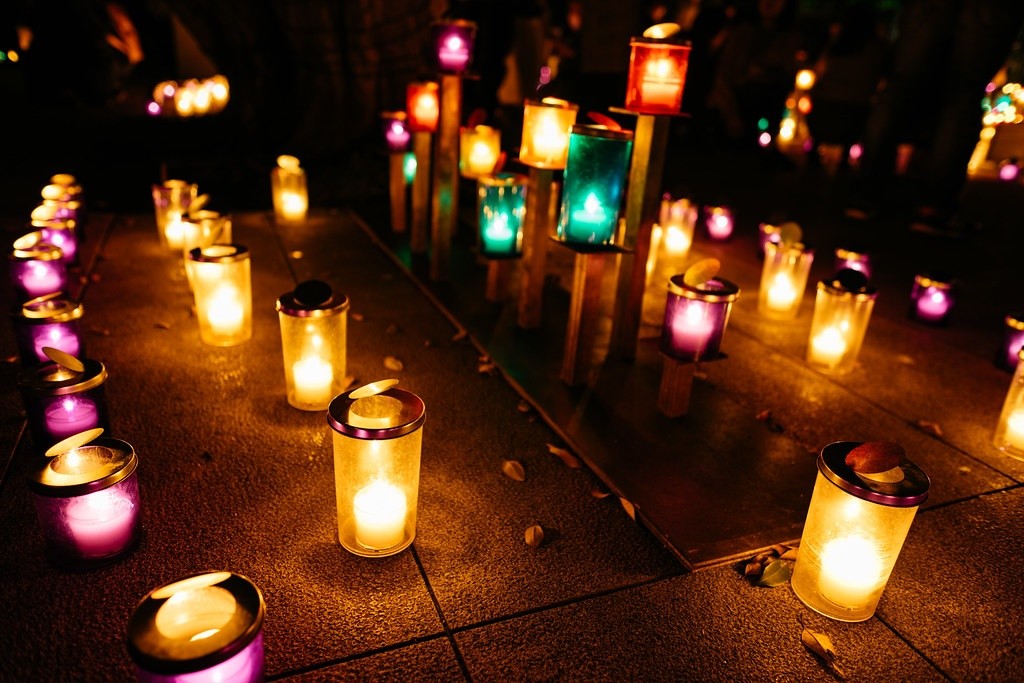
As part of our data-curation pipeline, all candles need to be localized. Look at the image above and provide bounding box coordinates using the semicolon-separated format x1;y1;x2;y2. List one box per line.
1004;409;1024;453
482;216;513;250
164;214;185;249
469;144;496;173
205;284;244;337
642;215;954;375
49;402;97;437
293;358;335;408
388;95;440;182
639;57;683;108
277;193;308;221
531;112;566;162
22;264;61;296
571;192;614;241
818;536;884;610
34;328;78;362
352;481;407;552
50;233;76;263
439;37;468;70
66;488;134;555
